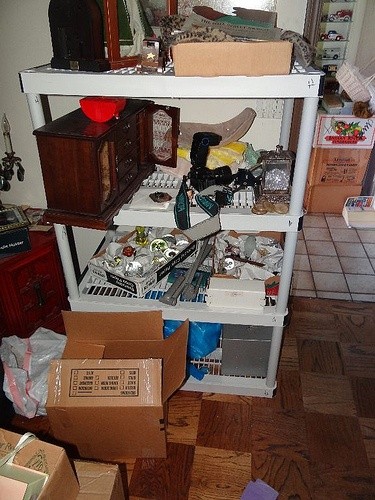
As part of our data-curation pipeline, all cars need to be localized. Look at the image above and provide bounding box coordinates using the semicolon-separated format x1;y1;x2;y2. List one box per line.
320;29;343;40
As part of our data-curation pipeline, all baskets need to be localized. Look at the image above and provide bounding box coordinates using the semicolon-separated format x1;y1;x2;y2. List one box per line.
335;55;375;104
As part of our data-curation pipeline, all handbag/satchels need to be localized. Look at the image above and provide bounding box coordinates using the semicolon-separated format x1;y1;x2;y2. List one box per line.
1;327;67;419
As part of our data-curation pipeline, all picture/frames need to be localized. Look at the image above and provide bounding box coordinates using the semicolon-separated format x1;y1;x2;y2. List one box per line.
0;206;31;232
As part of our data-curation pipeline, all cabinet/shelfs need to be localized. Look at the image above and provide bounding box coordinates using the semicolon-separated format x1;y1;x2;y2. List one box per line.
0;203;73;346
18;62;326;398
31;98;182;231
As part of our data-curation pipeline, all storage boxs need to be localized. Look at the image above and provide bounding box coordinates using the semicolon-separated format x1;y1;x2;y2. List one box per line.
0;428;80;500
88;224;198;297
311;106;375;149
66;455;127;500
303;179;363;214
44;310;190;458
172;38;294;76
307;148;372;186
220;321;275;377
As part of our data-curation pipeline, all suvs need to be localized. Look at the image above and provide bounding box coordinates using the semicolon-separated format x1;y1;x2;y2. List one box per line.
326;9;352;21
